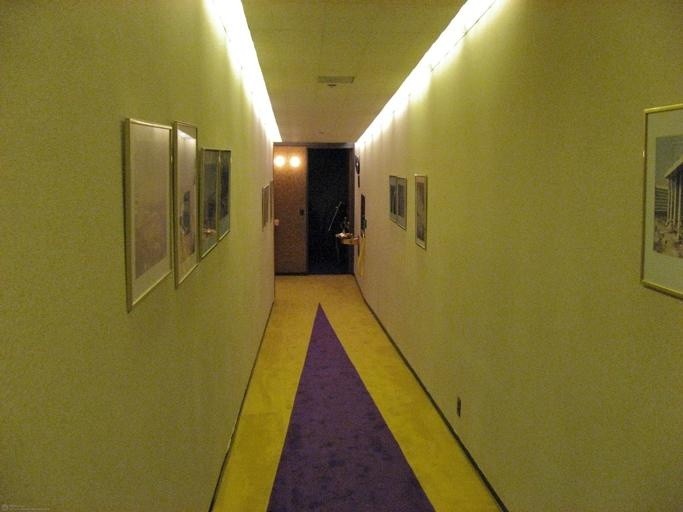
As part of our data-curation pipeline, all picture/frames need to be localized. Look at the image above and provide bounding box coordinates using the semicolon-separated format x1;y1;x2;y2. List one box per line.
262;179;274;230
387;174;429;252
638;104;683;302
122;118;232;317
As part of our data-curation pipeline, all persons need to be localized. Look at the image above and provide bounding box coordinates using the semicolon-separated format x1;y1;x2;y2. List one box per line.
179;191;195;258
